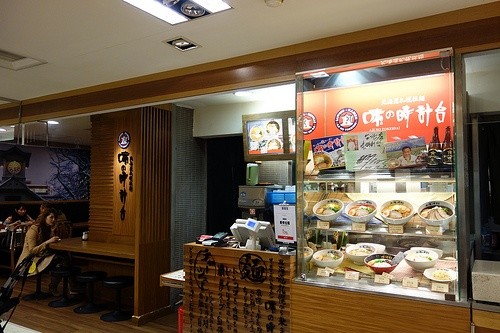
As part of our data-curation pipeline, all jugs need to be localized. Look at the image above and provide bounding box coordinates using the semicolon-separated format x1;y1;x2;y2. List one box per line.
246;163;258;186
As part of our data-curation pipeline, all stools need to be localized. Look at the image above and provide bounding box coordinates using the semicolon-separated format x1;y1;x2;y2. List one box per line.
104;275;134;321
73;271;108;314
47;267;83;308
22;265;53;300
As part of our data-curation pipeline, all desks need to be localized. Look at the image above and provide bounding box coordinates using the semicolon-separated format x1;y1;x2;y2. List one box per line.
0;227;25;234
48;222;135;268
159;268;186;288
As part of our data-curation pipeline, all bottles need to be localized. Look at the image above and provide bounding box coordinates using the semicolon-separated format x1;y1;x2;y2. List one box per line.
427;127;442;168
85;232;88;239
442;127;455;168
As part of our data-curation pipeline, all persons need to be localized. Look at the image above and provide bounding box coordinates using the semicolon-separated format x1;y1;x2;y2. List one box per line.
397;146;417;166
4;202;66;297
348;138;355;151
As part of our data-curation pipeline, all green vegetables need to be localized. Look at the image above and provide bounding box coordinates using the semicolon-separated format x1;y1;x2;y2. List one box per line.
442;208;449;213
329;206;340;212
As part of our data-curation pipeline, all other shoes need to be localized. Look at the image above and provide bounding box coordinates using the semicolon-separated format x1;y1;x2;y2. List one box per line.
68;292;86;300
48;287;63;297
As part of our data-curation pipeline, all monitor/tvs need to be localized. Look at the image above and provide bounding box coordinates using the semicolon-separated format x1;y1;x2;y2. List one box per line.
235;219;276;249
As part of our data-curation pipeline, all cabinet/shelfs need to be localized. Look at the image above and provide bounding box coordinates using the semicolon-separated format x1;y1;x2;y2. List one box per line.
297;172;460;301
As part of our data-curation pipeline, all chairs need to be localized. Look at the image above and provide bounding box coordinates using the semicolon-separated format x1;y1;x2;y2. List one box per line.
0;219;74;273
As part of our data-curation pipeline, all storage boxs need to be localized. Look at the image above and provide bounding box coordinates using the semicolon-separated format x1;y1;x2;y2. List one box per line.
268;191;296;243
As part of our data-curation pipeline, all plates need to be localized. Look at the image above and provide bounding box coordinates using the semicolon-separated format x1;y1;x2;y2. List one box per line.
423;267;457;282
259;182;274;186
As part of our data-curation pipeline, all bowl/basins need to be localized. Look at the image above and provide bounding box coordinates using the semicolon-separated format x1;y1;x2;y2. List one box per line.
314;152;333;170
403;249;439;272
313;198;344;222
364;253;399;275
304;246;313;264
313;249;344;268
418;201;455;227
379;199;414;225
345;200;377;222
304;199;308;214
358;242;386;254
346;244;376;265
